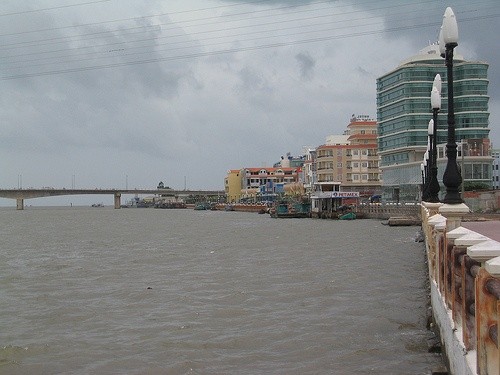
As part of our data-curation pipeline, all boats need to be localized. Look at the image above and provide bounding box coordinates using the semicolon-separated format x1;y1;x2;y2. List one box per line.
92;204;104;207
154;198;186;209
124;187;151;208
333;202;357;219
268;195;312;219
389;216;417;226
193;202;217;210
224;203;233;211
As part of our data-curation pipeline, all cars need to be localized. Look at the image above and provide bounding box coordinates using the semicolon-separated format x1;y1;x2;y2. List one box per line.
369;194;382;203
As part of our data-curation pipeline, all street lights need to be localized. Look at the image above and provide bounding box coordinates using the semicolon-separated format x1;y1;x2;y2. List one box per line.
421;73;441;203
441;8;466;205
17;172;187;191
230;193;258;205
